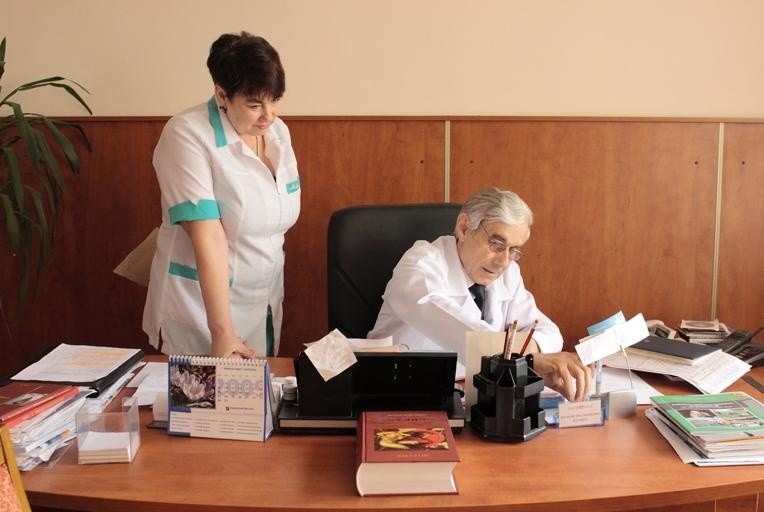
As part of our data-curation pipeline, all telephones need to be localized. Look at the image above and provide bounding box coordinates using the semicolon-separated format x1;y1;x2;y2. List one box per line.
714;324;763;366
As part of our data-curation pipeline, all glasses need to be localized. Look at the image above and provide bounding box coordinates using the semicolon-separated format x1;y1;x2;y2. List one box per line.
480;223;523;260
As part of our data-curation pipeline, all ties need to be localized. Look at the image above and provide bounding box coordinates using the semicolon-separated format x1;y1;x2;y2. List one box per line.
469;284;485;320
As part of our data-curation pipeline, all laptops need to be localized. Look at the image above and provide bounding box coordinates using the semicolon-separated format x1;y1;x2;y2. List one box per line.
293;352;457;417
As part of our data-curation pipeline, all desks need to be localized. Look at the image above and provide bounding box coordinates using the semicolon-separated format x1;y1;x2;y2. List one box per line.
0;355;764;512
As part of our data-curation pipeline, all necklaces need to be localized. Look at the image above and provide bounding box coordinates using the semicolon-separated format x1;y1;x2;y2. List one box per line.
256;135;260;159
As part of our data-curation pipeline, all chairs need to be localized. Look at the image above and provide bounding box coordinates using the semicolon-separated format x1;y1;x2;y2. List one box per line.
327;204;460;338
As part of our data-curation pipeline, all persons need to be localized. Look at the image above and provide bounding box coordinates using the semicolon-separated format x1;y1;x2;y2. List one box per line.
368;187;595;402
141;32;301;357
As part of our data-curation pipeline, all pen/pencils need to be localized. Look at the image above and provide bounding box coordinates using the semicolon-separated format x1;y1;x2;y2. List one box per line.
503;320;539;360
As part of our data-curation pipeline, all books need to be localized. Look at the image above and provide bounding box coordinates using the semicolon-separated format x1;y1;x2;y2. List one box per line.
644;391;764;467
354;410;461;496
625;321;734;382
0;342;143;471
